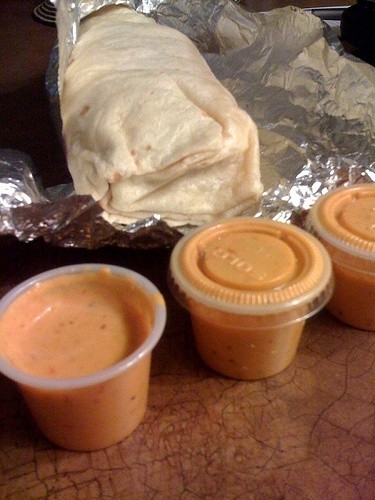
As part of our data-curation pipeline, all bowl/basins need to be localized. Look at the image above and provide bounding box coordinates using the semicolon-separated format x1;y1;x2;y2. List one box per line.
310;182;375;332
1;263;166;452
167;216;336;380
